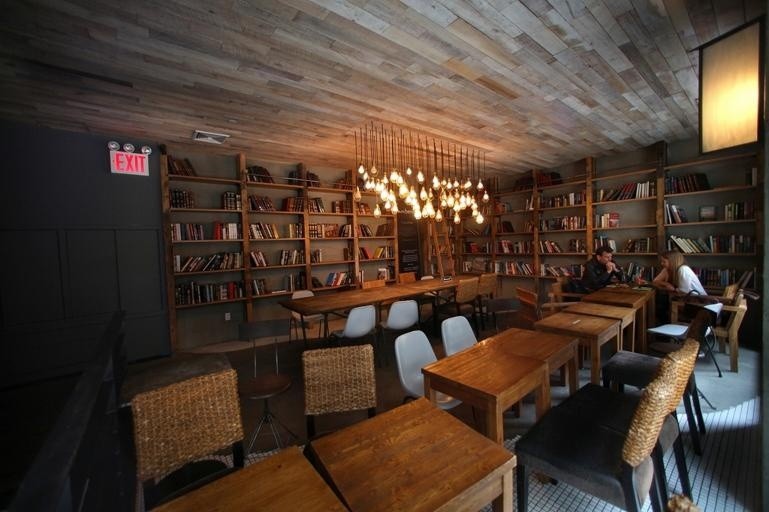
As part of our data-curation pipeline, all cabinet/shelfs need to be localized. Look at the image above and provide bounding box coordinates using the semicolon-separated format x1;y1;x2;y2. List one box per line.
661;141;764;294
243;150;309;346
356;172;394;285
159;151;247;358
536;160;589;299
421;184;454;273
490;169;537;298
589;140;661;286
456;180;492;274
307;155;360;290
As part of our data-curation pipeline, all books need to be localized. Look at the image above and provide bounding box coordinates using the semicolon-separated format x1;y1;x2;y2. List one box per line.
248;194;276;212
375;245;395;258
435;238;456;254
250;248;269;267
285;271;306;293
378;265;396;280
283;196;304;211
664;172;713;192
357;223;374;237
286;223;302;238
335;177;351;189
498;240;534;254
344;248;352;261
247;165;275;183
221;192;242;210
328;272;355;286
309;222;339;238
505;261;534;276
524;218;535;232
668;234;754;253
524;194;535;210
483;240;492;254
448;257;460;273
725;200;759;220
686;265;757;289
447;209;454;217
312;274;325;287
174;254;242;273
664;201;688;223
378;223;394;236
622;262;647;281
356;202;372;215
494;258;503;276
288;170;301;185
464;225;489;234
569;237;587;252
501;220;514;232
213;221;241;240
357;177;365;191
251;277;272;295
594;211;620;227
340;224;352;237
448;222;455;235
475;257;490;273
359;246;372;260
358;270;365;283
594;234;616;253
166;156;198;176
599;179;652;201
496;201;512;214
280;249;304;265
539;240;565;253
168;187;197;209
540;191;586;207
540;214;587;229
624;236;658;253
307;171;320;188
463;241;479;252
311;248;322;264
480;203;491;216
175;278;242;305
332;199;353;213
170;222;204;240
247;222;278;238
541;262;586;277
307;197;325;213
463;261;473;272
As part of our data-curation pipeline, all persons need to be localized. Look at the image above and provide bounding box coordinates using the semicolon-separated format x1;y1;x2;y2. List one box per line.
651;250;708;342
579;246;622;296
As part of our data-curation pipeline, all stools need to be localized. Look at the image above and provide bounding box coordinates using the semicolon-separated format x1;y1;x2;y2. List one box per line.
238;372;297;453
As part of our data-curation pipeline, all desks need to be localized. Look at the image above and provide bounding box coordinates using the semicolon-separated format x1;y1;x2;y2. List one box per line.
276;284;428;349
531;312;622;387
420;345;547;445
472;328;579;409
394;274;481;337
309;397;516;512
562;302;636;362
597;282;657;327
581;290;650;354
148;447;350;512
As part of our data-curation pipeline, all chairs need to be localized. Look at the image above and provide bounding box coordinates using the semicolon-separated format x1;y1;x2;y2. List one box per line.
601;338;706;456
288;291;323;342
361;280;385;289
670;298;747;373
130;369;244;512
329;305;375;339
557;351;695;510
673;289;743;353
646;302;722;377
437;277;479;337
478;274;496;316
514;376;683;511
514;286;543;322
670;284;737;316
394;330;463;410
440;316;477;356
398;272;415;284
379;300;418;347
301;343;377;438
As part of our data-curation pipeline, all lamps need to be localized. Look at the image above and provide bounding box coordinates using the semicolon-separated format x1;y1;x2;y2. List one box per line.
698;16;763;157
351;116;491;225
